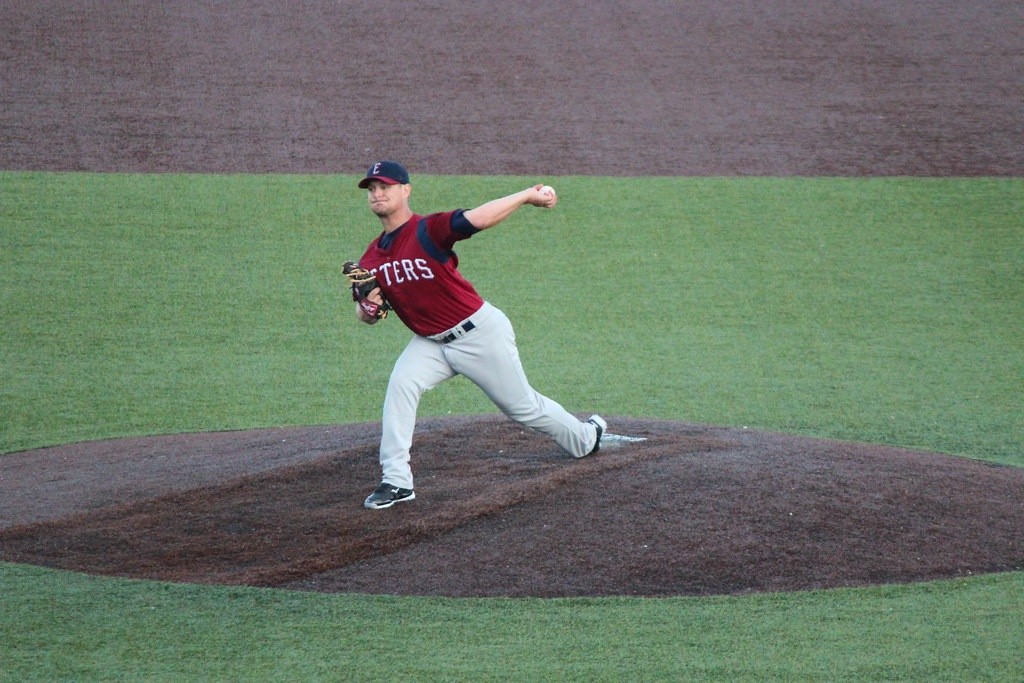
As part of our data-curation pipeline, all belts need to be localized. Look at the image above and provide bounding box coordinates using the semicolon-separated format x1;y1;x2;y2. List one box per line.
434;321;476;344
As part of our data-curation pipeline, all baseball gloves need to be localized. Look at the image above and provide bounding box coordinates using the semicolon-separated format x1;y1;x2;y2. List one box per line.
341;261;391;321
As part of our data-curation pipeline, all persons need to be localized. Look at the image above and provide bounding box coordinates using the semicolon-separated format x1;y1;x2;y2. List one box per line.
342;160;608;509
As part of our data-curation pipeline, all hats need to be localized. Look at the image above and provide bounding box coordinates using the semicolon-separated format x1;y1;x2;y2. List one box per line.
358;161;409;189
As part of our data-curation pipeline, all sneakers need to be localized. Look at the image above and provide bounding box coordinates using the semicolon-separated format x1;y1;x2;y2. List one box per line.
364;482;415;509
586;414;607;456
